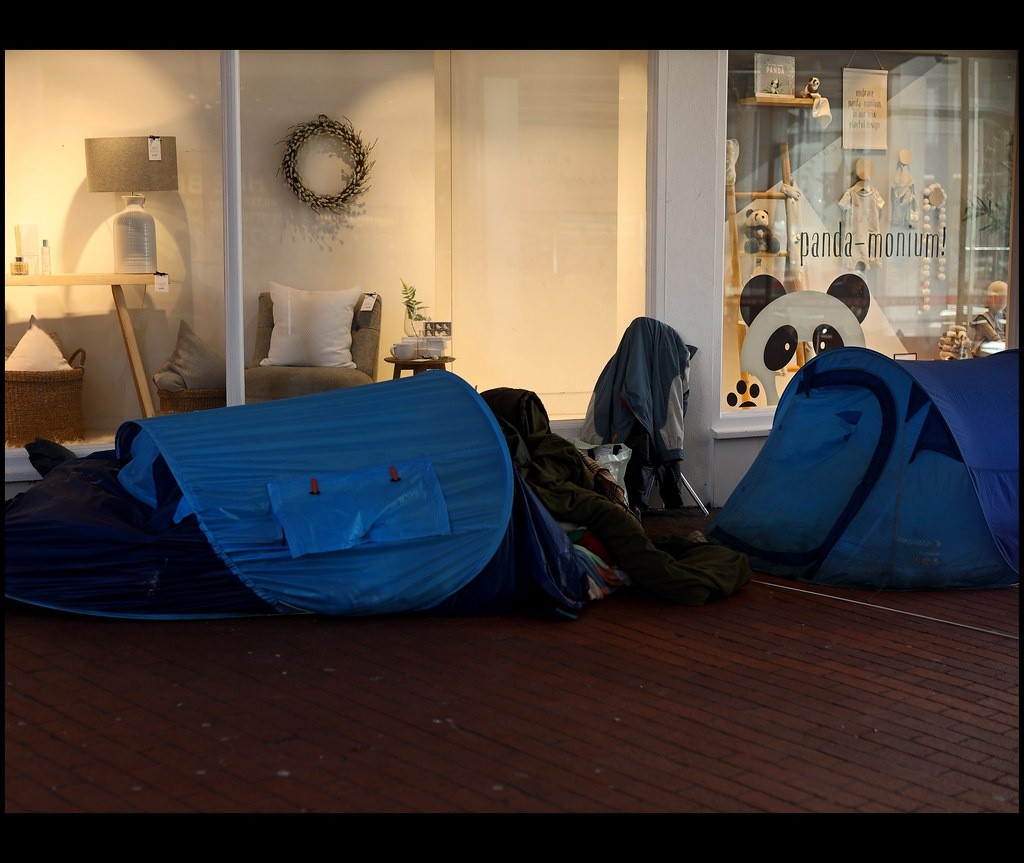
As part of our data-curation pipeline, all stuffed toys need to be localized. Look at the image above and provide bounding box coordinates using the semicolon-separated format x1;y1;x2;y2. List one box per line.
743;208;780;254
803;77;820;97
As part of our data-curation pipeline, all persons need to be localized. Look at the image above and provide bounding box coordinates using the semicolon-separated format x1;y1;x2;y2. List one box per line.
968;280;1008;358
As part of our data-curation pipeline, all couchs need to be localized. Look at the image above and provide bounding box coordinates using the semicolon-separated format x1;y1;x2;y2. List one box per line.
231;292;385;404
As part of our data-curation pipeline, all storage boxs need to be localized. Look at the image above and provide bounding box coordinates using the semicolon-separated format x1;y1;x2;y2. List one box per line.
3;347;90;447
154;370;228;414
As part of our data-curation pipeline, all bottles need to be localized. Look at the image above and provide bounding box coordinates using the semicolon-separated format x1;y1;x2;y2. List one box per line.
113;195;158;274
41;238;52;274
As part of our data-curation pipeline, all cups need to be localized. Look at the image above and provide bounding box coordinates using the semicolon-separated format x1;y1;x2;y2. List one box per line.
389;343;415;360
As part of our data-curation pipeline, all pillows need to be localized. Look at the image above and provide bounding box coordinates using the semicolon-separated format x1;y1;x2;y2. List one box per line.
256;281;364;369
5;315;75;371
152;319;226;392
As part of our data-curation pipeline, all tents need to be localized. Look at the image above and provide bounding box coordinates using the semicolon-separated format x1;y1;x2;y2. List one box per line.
4;314;751;623
707;347;1020;592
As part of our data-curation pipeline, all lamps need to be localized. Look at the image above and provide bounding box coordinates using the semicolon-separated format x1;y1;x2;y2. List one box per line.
88;133;180;270
